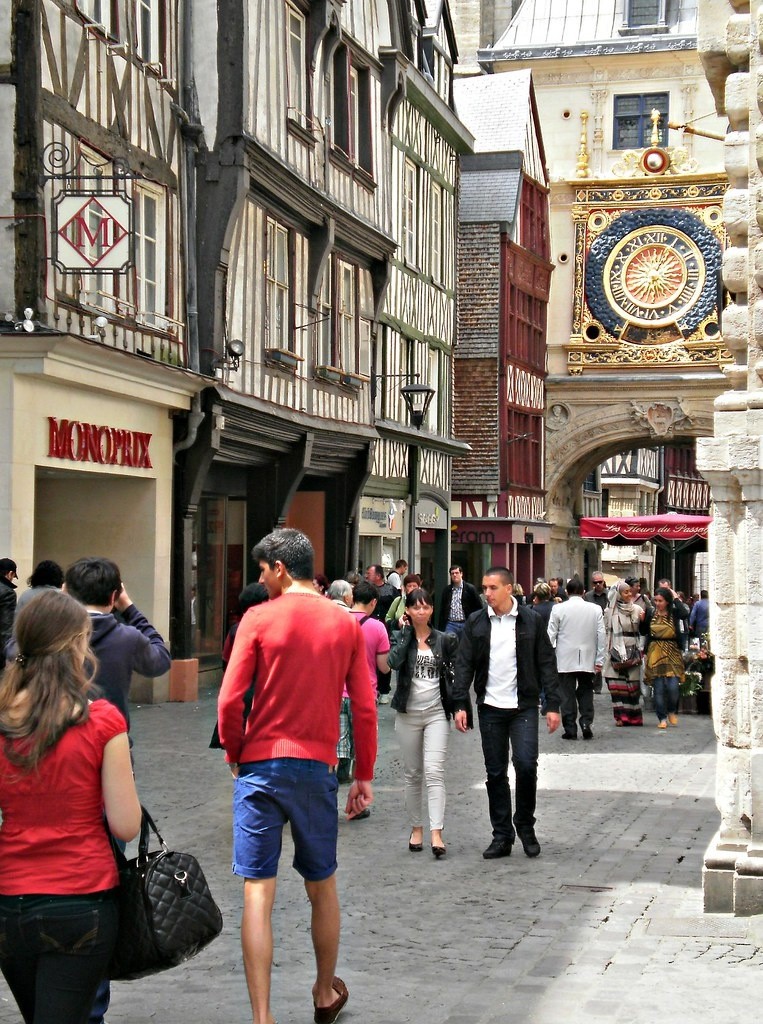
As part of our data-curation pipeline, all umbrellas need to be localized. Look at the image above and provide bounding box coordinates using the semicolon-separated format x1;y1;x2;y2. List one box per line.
578;512;714;595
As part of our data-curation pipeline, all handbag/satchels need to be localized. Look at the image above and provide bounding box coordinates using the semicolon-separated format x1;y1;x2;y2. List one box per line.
609;644;643;672
102;802;223;981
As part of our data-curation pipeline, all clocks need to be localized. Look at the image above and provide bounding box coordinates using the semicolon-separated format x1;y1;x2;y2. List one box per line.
602;225;705;328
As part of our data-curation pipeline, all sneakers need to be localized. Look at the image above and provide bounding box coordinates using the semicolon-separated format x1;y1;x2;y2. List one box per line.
483;839;512;858
516;827;541;858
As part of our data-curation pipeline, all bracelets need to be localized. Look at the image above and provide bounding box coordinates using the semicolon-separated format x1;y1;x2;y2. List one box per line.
226;763;238;769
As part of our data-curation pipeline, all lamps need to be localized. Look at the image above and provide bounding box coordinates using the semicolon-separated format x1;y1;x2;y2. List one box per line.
371;373;436;431
5;308;35;332
372;498;397;512
224;340;245;372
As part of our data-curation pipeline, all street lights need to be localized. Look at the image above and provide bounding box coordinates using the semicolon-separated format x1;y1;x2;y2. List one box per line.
399;382;436;578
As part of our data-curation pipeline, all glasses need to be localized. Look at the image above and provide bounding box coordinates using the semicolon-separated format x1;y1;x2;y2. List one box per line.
593;581;603;584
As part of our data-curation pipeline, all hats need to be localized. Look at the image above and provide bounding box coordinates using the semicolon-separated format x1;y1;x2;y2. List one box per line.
0;558;18;580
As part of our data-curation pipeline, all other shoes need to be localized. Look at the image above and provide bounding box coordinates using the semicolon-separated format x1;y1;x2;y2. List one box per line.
431;842;447;859
668;714;678;725
409;831;422;852
313;975;349;1024
338;775;352;784
657;722;668;729
378;693;390;704
562;732;578;740
595;689;601;694
582;723;593;739
540;712;547;717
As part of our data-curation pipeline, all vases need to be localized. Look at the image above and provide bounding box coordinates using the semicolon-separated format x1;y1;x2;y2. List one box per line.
681;696;698;715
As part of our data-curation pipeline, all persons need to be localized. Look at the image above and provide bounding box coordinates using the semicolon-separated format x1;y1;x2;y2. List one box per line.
190;589;197;651
0;558;17;669
308;560;708;738
13;559;63;641
217;527;377;1024
334;580;390;819
453;566;561;859
0;590;142;1024
64;558;171;1024
386;589;473;856
222;583;269;730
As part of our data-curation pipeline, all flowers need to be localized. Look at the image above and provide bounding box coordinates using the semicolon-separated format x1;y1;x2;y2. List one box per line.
679;671;703;696
689;632;708;662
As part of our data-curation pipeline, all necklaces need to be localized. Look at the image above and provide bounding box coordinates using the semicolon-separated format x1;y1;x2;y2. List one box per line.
416;635;421;643
